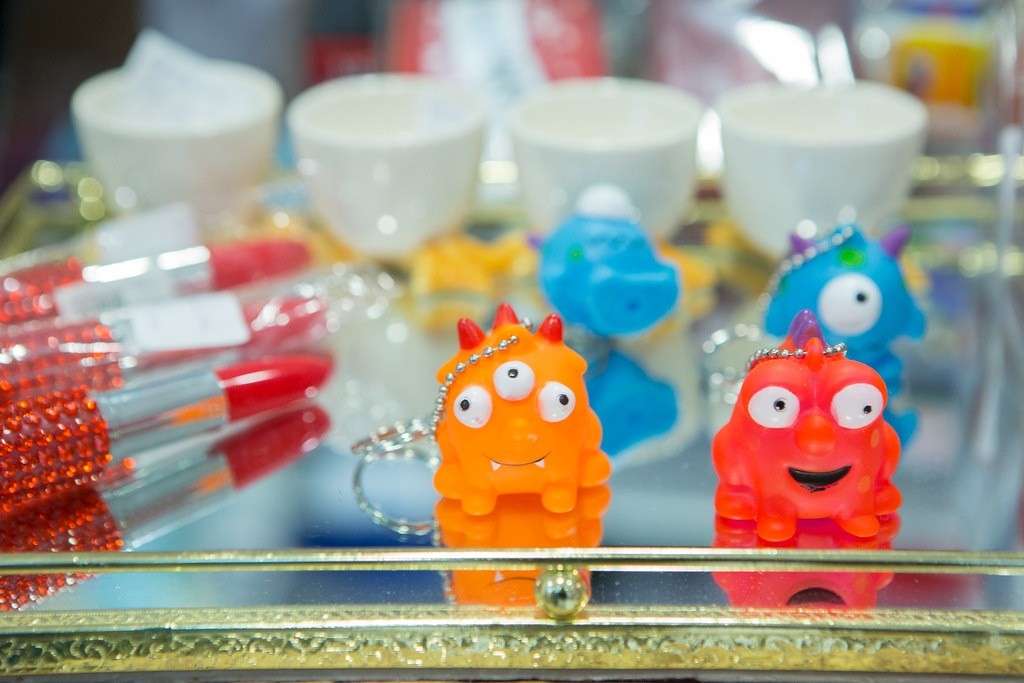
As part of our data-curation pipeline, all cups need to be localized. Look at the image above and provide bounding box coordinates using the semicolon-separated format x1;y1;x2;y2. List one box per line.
286;71;489;258
723;79;928;258
508;78;699;243
72;60;282;220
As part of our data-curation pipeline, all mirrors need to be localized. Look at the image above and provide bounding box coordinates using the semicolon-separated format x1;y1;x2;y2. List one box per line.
0;0;1024;683
